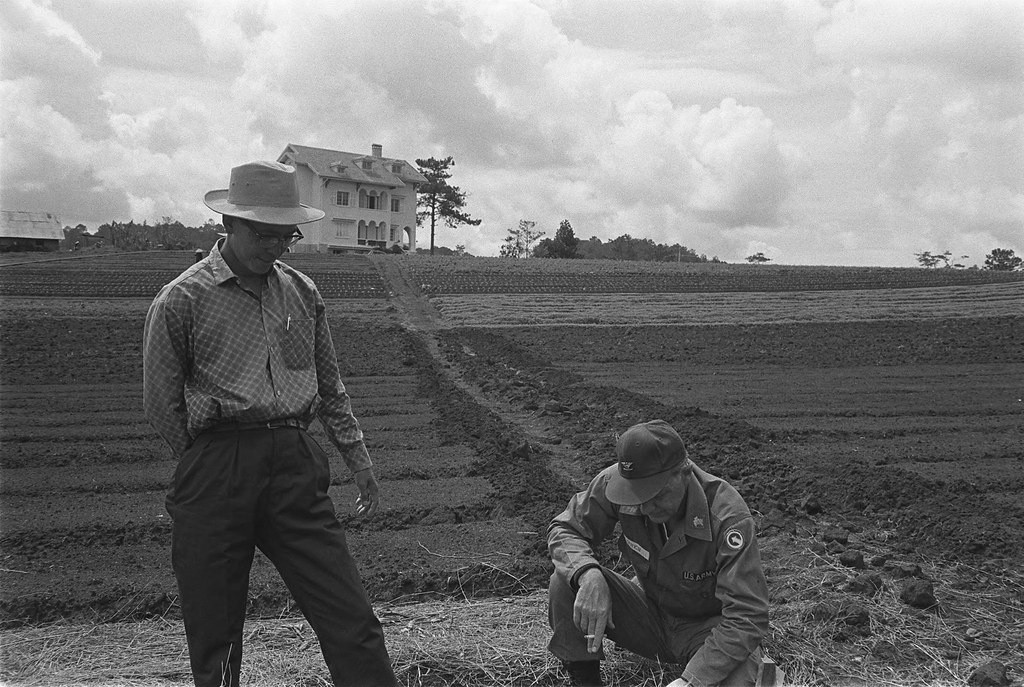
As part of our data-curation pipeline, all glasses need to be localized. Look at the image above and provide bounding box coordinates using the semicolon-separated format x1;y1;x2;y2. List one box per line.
241;220;305;249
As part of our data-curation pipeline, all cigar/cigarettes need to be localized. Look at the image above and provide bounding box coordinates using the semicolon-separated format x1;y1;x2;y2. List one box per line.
584;634;607;639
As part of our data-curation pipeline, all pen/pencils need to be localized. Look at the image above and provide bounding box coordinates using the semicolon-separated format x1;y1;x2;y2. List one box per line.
286;314;292;332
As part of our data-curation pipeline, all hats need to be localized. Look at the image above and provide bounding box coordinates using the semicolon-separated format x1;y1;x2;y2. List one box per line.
606;419;686;506
204;159;325;225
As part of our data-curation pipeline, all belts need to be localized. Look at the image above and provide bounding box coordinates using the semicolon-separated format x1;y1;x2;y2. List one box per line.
208;417;306;435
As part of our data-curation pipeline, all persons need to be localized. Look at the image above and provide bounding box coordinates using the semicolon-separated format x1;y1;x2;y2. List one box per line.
141;159;399;687
545;419;769;687
